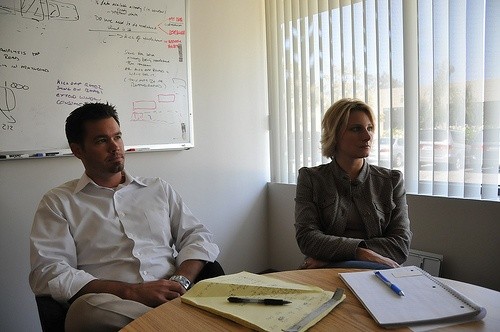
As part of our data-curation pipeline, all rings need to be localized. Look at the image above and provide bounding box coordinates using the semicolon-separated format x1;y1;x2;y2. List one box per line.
305;262;308;264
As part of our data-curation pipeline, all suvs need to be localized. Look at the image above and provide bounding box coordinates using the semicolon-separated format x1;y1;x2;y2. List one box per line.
419;127;472;170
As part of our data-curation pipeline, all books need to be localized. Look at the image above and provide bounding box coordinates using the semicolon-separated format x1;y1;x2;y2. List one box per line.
338;266;488;332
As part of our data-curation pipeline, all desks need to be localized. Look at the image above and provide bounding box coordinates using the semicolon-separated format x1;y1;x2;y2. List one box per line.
117;269;500;332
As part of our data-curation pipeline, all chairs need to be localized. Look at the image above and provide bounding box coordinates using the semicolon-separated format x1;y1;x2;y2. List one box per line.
35;262;224;332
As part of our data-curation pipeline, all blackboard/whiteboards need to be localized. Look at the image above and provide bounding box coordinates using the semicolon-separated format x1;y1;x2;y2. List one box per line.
0;0;195;162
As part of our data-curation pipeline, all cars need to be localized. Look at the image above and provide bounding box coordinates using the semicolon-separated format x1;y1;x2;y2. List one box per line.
370;136;404;167
469;128;500;173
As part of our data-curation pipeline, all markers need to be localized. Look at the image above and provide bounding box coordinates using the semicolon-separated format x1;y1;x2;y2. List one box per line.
127;148;150;151
0;152;59;159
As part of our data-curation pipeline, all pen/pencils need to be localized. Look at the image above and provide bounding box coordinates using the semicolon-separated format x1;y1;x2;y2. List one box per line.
374;271;405;296
227;296;292;305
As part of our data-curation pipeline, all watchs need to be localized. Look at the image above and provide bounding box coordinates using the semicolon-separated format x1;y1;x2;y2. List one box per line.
169;275;192;290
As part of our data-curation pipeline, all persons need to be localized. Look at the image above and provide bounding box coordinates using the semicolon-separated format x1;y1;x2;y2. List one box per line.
28;102;220;332
294;98;412;269
